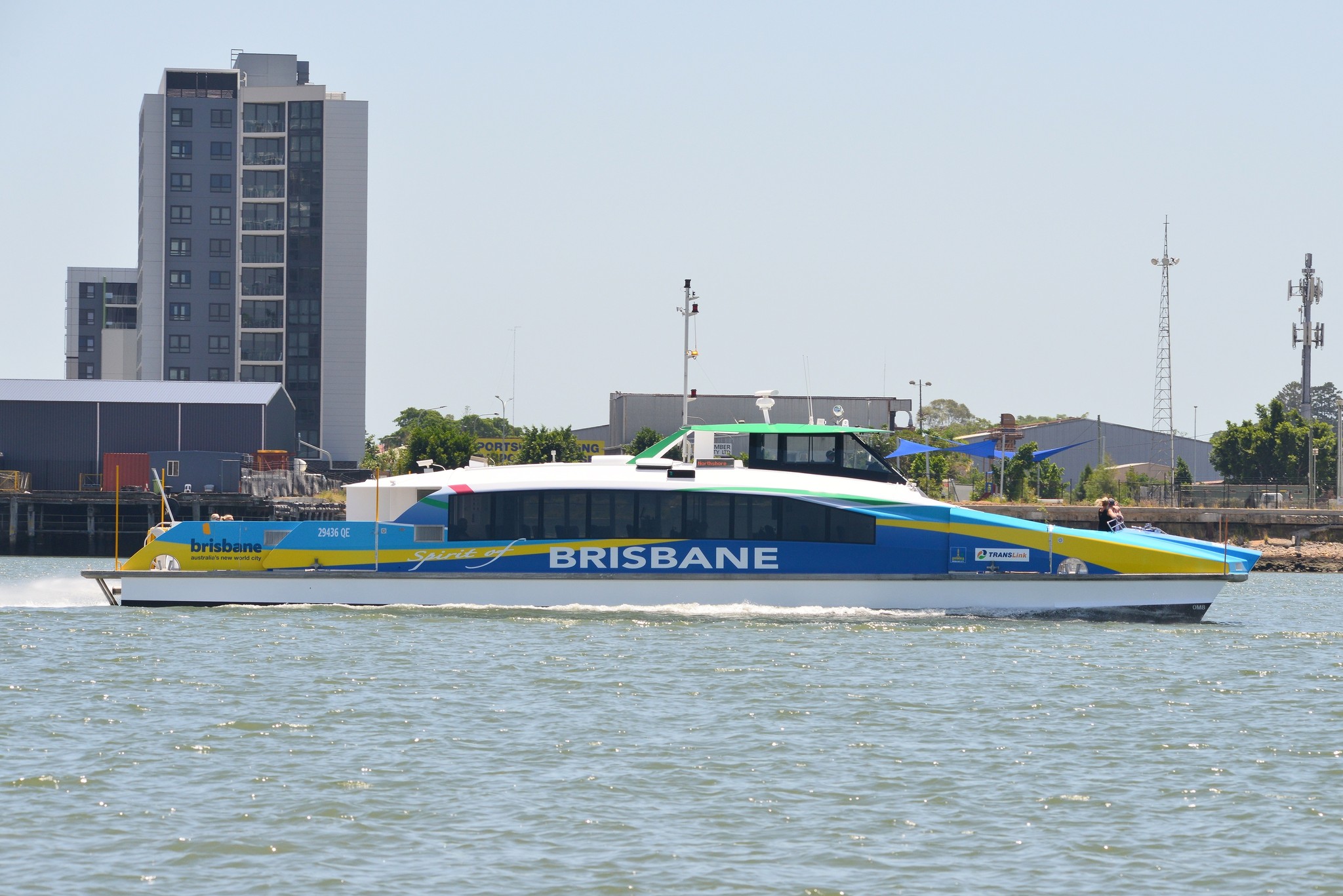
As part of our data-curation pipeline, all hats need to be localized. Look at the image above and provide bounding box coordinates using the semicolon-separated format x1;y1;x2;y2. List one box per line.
1109;499;1115;505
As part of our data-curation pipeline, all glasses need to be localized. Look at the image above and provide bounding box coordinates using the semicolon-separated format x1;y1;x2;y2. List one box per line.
216;518;220;520
228;519;234;521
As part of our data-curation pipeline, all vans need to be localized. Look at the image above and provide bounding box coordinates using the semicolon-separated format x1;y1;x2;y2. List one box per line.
1261;493;1285;507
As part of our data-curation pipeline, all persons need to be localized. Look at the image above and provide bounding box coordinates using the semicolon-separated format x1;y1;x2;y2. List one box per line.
211;514;220;521
825;451;835;462
1098;497;1124;531
220;515;234;521
689;518;708;537
457;518;469;537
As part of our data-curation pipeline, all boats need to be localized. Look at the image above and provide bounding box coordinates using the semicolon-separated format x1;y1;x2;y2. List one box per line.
78;280;1262;626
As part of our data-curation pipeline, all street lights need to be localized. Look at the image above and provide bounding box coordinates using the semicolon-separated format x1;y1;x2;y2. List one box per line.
418;405;447;428
382;436;401;453
909;379;932;430
495;395;513;463
1194;406;1197;484
474;413;499;432
